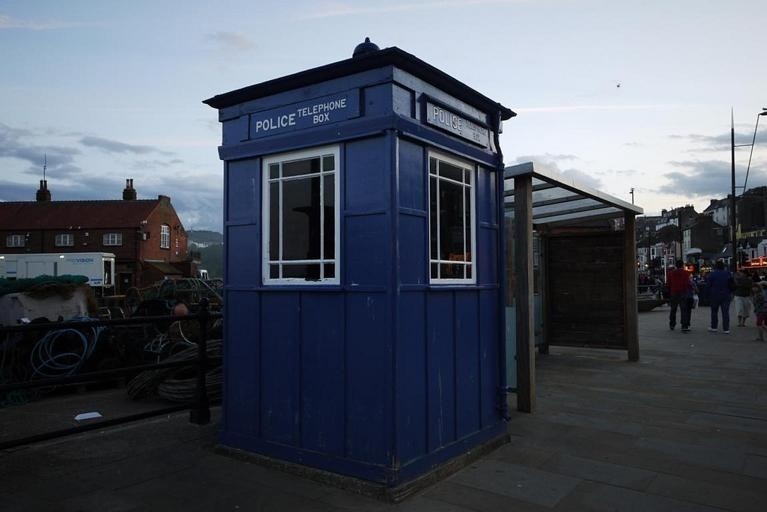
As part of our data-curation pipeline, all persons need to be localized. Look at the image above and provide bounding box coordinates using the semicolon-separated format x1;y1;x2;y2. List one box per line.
705;260;733;334
666;259;690;331
733;264;766;342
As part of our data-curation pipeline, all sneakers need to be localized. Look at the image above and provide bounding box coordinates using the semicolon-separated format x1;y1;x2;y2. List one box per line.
681;328;690;331
752;337;763;342
707;327;730;334
